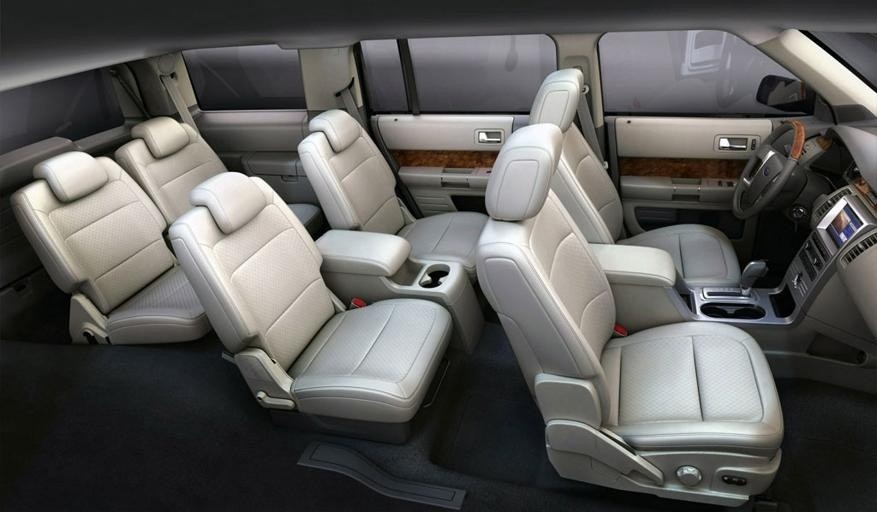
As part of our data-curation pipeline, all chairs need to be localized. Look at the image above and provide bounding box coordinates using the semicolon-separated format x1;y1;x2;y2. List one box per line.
9;69;785;507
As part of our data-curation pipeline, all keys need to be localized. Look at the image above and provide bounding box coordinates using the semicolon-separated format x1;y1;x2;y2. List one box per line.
791;208;805;232
